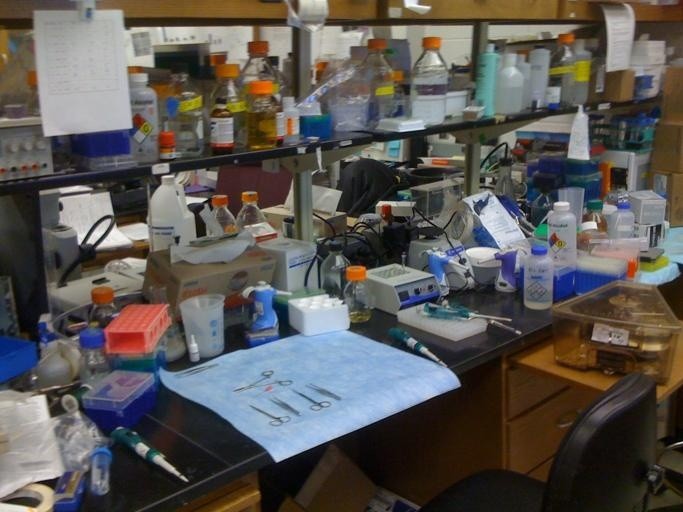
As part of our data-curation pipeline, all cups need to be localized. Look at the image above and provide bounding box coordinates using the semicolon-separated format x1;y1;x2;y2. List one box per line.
178;291;227;363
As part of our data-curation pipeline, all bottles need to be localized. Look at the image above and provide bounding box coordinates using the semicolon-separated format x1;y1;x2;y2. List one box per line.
546;184;635;279
127;39;302;166
320;241;352;303
496;156;517;205
79;328;111;391
343;265;371;323
206;193;237;238
89;448;113;494
89;285;119;330
361;32;604;125
523;244;554;311
236;190;267;236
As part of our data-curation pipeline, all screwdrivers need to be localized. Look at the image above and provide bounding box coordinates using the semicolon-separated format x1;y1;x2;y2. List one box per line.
423;301;523;336
388;327;450;368
111;425;189;483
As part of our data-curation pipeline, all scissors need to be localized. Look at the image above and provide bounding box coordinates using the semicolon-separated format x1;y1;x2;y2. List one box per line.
250;403;291;426
294;390;331;411
233;371;292;393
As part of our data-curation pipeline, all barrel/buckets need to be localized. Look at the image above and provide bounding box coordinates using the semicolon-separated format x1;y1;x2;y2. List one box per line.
144;173;199;250
